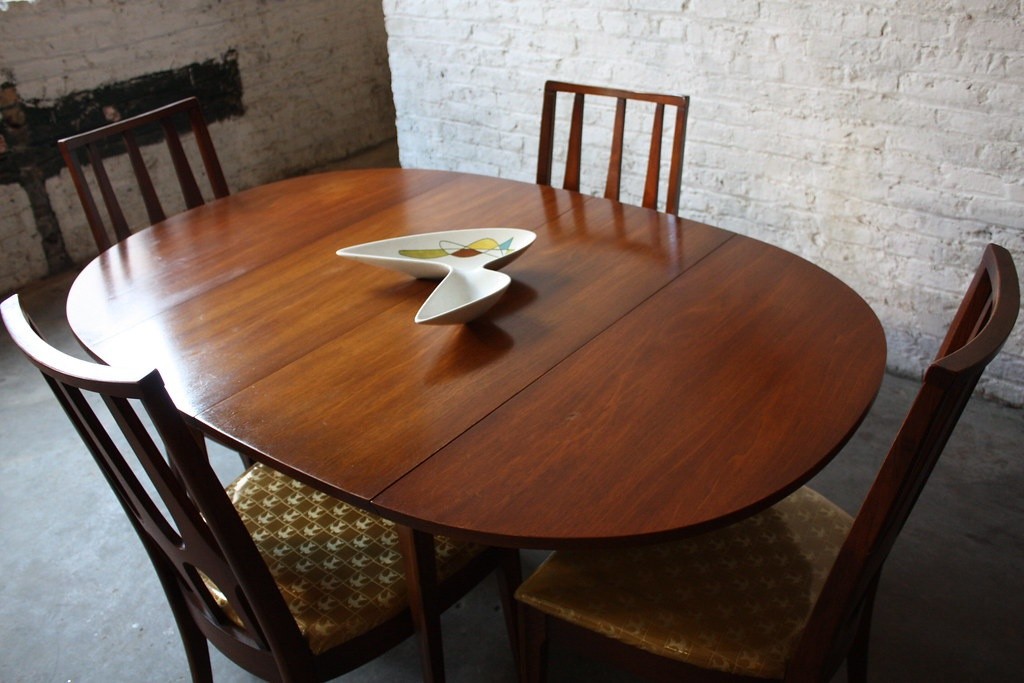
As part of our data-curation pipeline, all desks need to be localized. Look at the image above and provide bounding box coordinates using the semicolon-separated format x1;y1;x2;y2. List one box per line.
65;168;887;683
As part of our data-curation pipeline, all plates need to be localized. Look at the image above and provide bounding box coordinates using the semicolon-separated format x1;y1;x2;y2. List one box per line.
335;228;537;325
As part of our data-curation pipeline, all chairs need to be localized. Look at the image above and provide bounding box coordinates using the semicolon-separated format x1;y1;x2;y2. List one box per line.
0;293;523;683
513;243;1020;683
57;96;257;476
535;80;690;215
